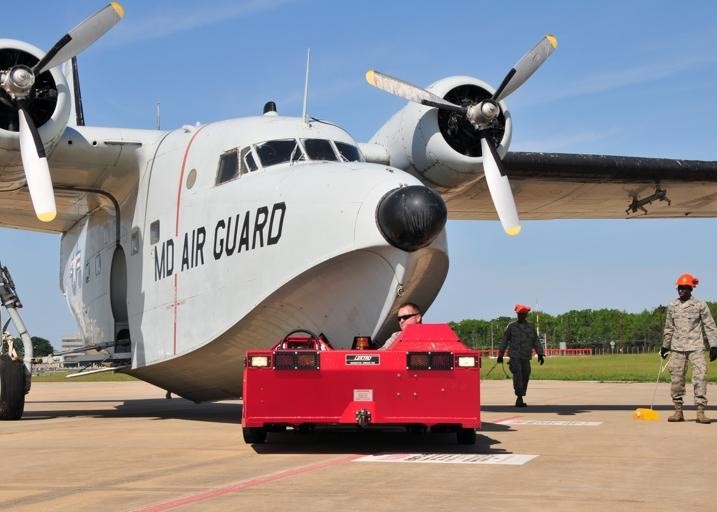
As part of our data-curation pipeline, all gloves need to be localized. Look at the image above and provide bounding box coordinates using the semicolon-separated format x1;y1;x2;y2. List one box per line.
709;347;717;361
661;346;669;359
538;355;544;365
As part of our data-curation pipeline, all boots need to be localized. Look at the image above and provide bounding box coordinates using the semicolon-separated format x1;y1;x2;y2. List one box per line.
668;401;683;421
696;406;711;423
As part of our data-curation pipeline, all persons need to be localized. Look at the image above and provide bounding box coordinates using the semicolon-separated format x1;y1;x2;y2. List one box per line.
658;273;716;424
375;302;422;350
497;304;544;408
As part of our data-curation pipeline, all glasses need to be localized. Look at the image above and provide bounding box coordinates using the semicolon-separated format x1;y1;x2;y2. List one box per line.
397;313;417;321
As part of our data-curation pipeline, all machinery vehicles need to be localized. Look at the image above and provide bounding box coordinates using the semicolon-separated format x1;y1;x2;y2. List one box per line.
240;322;483;447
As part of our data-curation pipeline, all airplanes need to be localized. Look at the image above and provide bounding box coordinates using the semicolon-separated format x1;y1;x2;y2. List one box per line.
0;0;717;422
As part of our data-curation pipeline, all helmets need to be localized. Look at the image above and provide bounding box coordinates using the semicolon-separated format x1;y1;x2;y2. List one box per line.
676;274;696;287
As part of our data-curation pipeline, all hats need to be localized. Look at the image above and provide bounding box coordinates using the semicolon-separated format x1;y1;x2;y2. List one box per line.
514;305;531;313
